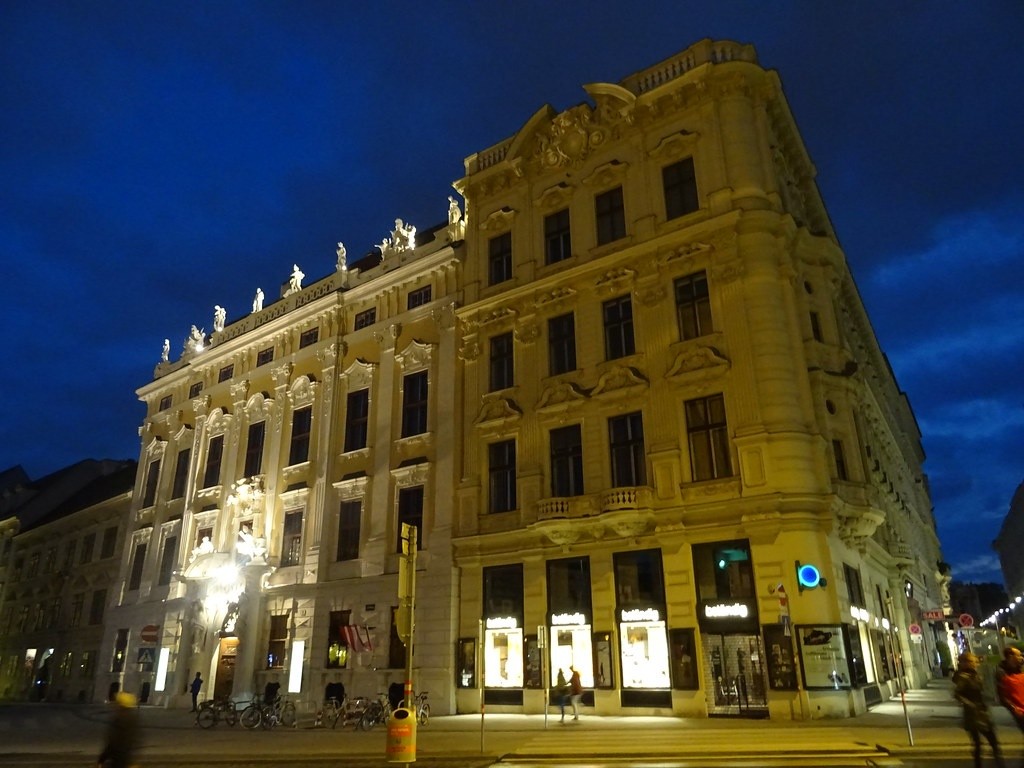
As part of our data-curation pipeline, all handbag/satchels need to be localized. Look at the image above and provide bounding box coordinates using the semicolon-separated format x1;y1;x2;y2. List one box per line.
559;686;572;697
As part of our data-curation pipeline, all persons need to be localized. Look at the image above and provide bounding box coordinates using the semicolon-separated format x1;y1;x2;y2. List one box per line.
252;288;265;314
558;665;583;722
448;195;461;224
189;672;203;712
950;650;1004;768
336;241;347;270
214;305;226;333
161;339;169;362
289;264;304;290
994;647;1024;732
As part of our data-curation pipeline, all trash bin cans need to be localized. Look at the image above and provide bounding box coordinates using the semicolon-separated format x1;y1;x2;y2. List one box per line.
386;708;416;762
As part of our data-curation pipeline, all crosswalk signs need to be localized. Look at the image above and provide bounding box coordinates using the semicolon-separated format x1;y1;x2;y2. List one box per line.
137;647;157;663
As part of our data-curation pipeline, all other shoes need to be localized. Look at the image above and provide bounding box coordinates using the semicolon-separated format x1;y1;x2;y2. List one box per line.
190;709;196;713
572;717;578;721
559;721;564;723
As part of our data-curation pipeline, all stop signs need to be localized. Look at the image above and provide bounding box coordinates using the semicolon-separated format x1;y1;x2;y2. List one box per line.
141;626;159;642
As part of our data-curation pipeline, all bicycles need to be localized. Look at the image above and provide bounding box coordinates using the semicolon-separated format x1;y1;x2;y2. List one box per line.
193;689;430;731
